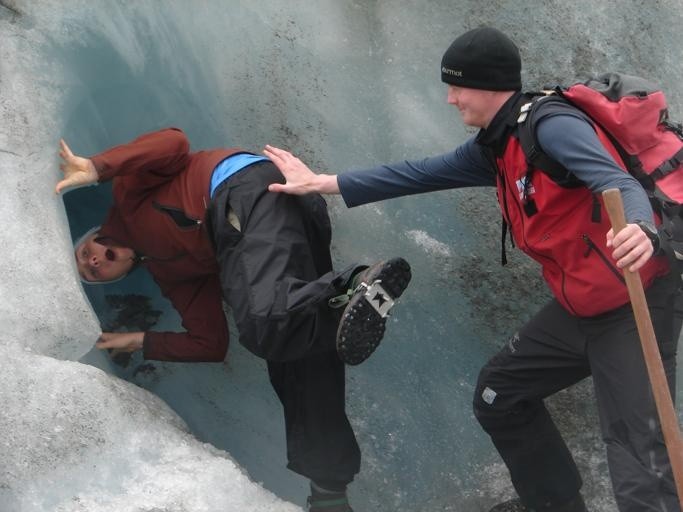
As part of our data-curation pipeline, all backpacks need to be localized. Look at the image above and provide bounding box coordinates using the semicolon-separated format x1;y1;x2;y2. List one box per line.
516;71;682;279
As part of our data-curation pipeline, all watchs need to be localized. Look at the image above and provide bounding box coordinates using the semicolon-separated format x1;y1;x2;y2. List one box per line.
638;219;662;257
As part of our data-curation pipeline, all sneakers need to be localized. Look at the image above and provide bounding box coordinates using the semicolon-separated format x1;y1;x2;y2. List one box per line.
329;257;412;365
486;497;589;512
306;482;354;512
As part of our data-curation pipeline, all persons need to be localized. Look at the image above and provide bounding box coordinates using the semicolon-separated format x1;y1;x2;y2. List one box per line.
259;24;683;512
52;125;414;511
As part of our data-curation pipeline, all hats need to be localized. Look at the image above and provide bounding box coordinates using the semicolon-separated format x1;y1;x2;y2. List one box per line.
74;226;128;284
441;28;522;90
